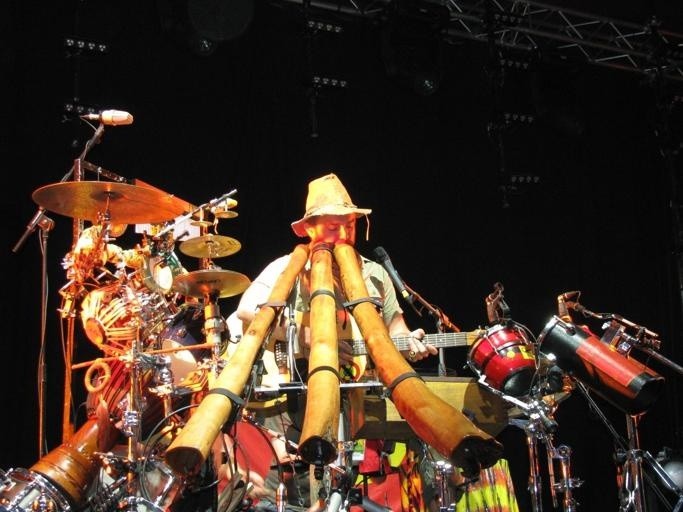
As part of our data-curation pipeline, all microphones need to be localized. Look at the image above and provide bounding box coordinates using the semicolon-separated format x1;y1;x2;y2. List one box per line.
78;109;133;125
373;246;413;304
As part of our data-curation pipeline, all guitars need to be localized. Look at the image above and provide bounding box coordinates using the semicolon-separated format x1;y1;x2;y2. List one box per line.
262;316;486;386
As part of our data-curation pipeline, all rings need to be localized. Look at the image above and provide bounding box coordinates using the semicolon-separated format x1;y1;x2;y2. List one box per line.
408;350;416;358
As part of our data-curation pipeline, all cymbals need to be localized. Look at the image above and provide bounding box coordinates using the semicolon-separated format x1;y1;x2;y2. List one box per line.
179;233;242;258
171;269;251;299
31;180;185;225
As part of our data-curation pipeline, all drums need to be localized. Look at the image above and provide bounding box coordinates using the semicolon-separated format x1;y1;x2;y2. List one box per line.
140;250;181;296
536;313;664;408
79;278;176;357
146;302;231;388
465;322;540;407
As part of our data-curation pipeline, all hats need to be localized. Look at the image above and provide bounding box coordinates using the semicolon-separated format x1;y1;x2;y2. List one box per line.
291;171;372;237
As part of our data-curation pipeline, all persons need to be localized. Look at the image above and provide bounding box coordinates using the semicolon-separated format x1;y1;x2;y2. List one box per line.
235;175;439;365
